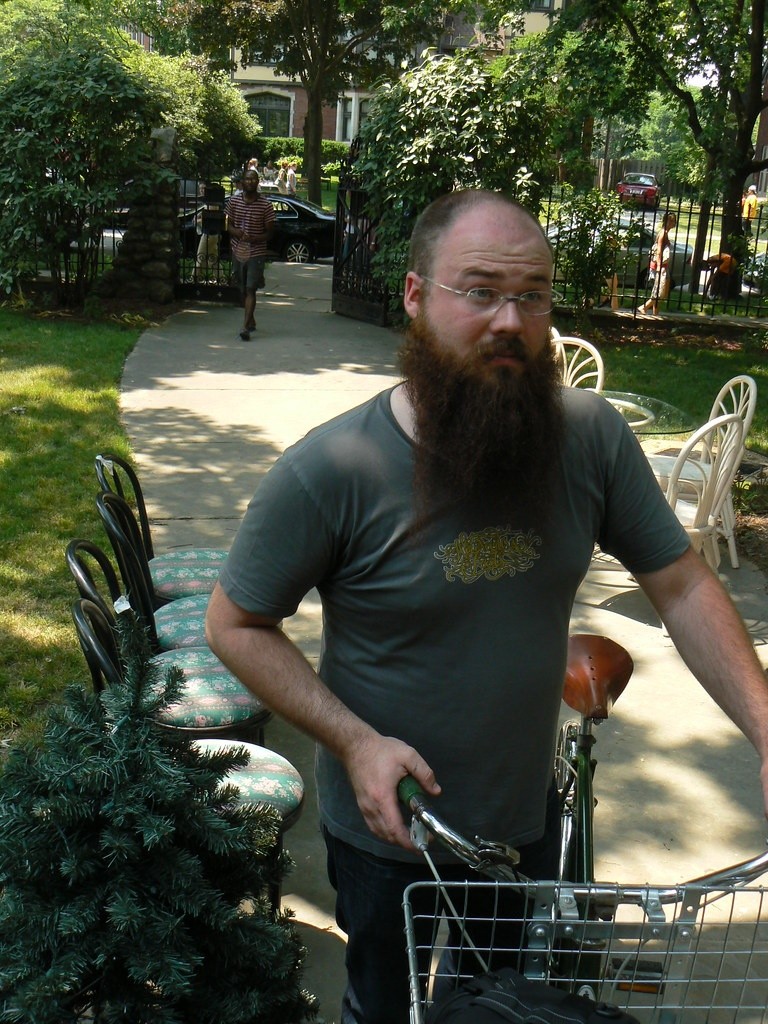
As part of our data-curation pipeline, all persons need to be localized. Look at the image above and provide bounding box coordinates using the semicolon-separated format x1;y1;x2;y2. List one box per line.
223;169;276;342
189;157;300;281
582;208;624;311
706;245;741;302
638;212;676;315
742;184;758;238
203;186;768;1024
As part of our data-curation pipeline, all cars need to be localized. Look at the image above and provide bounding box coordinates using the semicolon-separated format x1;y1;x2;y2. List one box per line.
104;178;230;230
742;253;768;292
178;192;347;264
541;216;693;290
616;173;663;212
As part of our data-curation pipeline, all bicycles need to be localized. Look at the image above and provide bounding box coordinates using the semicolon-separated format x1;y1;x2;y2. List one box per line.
396;633;768;1023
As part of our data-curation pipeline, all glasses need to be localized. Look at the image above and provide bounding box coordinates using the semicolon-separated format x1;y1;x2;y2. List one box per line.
420;275;563;316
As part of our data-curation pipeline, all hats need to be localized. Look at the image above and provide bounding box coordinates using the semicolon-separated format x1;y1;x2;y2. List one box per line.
749;185;756;193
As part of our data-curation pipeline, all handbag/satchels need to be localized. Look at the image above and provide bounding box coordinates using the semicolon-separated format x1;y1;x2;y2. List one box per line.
274;170;284;187
653;246;672;265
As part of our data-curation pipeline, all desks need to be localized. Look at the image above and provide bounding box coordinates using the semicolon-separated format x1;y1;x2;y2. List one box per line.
574;387;697;435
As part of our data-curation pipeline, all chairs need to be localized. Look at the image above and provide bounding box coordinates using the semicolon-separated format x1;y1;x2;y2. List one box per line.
651;413;743;637
637;375;757;569
544;323;605;391
67;451;306;922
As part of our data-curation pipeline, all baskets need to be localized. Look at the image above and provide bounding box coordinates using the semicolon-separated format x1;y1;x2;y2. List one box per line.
403;880;768;1024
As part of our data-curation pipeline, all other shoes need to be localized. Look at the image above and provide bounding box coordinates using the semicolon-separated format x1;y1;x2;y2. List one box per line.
239;325;257;341
